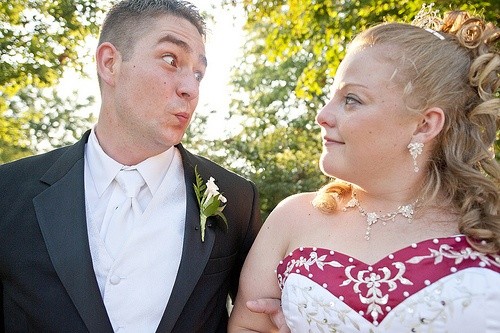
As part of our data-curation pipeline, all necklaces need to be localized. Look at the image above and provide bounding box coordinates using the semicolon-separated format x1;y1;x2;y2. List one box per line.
342;190;419;241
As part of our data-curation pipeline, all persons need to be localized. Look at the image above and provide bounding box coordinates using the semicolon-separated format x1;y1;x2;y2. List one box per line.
227;7;500;333
1;1;291;333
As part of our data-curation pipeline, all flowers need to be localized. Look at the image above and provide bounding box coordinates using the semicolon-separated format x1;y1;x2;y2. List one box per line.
193;164;229;242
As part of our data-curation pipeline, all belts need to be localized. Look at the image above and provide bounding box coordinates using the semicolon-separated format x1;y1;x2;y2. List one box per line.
104;170;146;261
